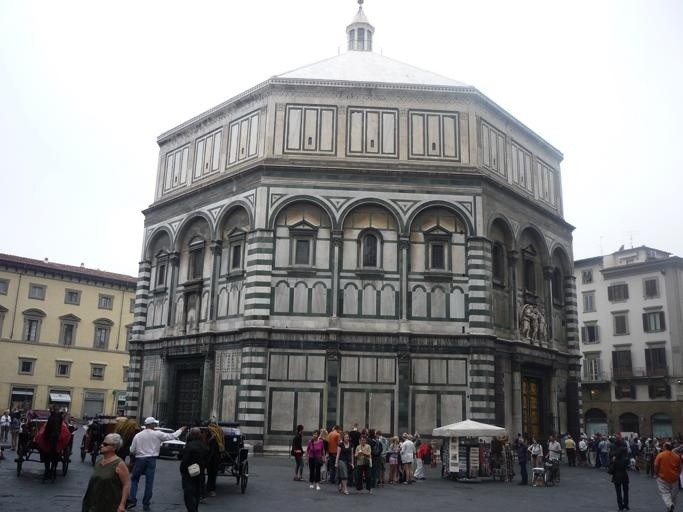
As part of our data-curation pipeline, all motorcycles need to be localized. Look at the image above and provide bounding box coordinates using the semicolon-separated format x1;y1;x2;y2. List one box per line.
543;457;562;487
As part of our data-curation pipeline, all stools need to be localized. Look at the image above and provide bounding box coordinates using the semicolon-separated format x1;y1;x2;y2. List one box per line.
530;467;548;488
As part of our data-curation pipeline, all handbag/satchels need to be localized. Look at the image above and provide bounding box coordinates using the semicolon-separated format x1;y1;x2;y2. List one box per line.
188;463;201;476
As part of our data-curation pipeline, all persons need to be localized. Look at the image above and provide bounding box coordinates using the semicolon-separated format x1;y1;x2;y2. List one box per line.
513;431;683;512
0;406;189;511
291;423;437;495
521;305;549;343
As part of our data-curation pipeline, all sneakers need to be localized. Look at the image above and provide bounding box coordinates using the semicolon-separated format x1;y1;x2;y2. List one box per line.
294;476;426;495
199;490;218;505
616;500;679;511
518;478;561;486
127;501;151;511
567;459;661;477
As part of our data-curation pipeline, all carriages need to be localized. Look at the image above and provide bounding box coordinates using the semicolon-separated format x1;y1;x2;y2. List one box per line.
80;414;141;472
14;406;79;484
178;416;250;503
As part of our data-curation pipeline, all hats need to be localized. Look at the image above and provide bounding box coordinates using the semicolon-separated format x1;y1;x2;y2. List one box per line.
144;417;159;424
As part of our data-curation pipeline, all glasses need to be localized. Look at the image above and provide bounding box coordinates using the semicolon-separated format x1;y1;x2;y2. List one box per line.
102;443;115;447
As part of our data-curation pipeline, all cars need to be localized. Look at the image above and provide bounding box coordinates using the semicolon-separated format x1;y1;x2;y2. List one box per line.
141;425;185;460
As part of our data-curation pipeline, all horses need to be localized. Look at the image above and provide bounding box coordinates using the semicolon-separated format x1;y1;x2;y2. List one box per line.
112;418;143;473
39;406;66;484
195;427;219;504
